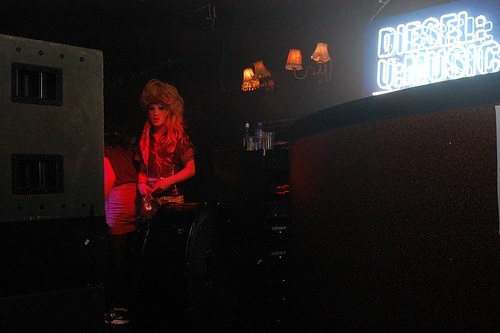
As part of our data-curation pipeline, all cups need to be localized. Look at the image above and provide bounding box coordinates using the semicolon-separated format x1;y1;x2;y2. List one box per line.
264;132;272;149
246;137;255;150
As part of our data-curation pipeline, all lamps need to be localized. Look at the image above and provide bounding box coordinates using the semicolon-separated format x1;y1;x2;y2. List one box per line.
241;42;332;96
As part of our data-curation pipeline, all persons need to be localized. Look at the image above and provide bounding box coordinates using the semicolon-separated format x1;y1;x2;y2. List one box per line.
138;78;197;222
104;141;141;324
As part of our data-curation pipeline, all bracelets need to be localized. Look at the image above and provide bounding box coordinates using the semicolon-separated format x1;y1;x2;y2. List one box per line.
138;182;146;185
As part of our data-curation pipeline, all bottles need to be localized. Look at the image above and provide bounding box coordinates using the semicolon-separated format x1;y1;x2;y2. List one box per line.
188;196;194;202
243;123;251;146
255;122;264;150
